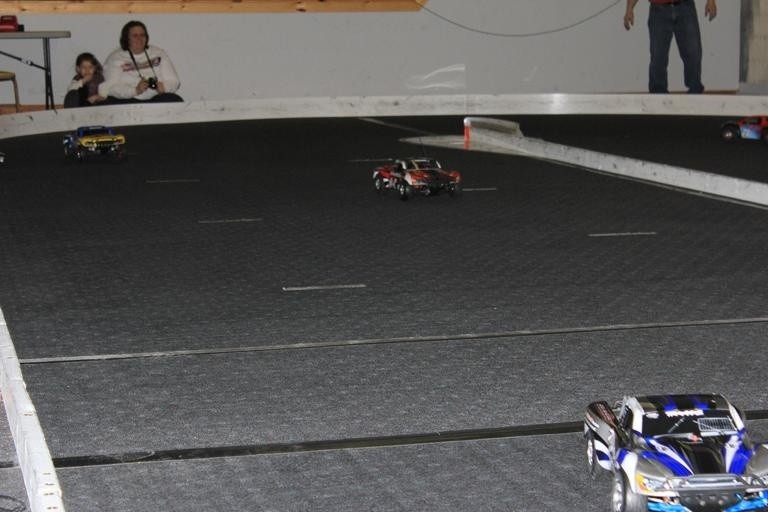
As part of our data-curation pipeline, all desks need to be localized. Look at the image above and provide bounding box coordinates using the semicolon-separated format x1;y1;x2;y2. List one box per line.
0;30;71;111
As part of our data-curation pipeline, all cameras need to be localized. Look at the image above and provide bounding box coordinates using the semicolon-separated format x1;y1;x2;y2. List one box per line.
145;77;158;89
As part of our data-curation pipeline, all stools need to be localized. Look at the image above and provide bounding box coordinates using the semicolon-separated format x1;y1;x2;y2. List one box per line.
0;71;20;113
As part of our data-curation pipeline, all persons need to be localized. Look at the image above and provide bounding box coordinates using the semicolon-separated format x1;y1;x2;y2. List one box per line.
623;1;718;93
101;20;187;102
63;52;113;107
